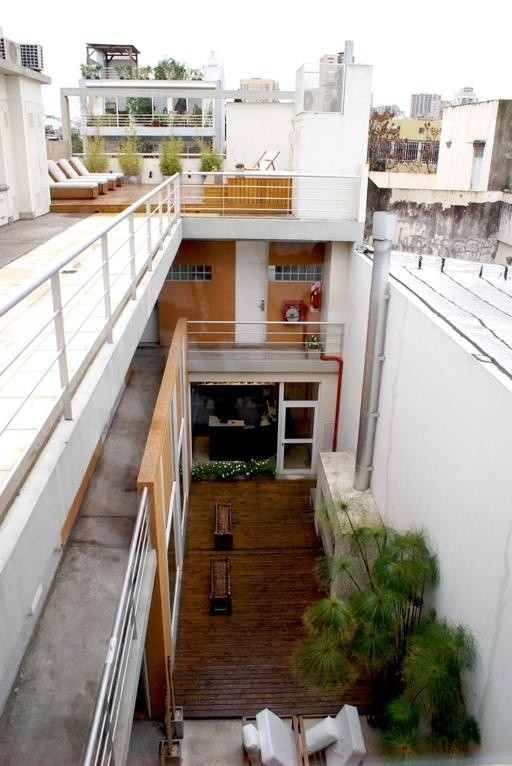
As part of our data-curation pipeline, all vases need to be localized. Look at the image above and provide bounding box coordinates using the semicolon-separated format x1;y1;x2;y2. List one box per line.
304;340;321;360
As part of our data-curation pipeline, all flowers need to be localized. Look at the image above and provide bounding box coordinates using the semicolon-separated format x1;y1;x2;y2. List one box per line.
191;458;276;481
306;334;319;349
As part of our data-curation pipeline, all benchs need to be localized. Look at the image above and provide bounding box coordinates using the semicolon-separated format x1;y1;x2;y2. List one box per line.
214;504;234;550
209;557;233;617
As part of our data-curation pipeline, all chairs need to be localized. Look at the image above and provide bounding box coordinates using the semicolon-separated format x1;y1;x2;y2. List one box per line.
46;155;125;198
245;149;281;172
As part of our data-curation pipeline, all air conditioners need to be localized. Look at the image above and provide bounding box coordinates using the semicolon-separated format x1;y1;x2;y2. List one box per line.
0;34;44;71
317;53;355;87
303;86;331;112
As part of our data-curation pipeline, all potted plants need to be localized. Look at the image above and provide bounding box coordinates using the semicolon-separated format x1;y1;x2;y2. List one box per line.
117;133;181;185
202;150;223;184
235;162;245;177
